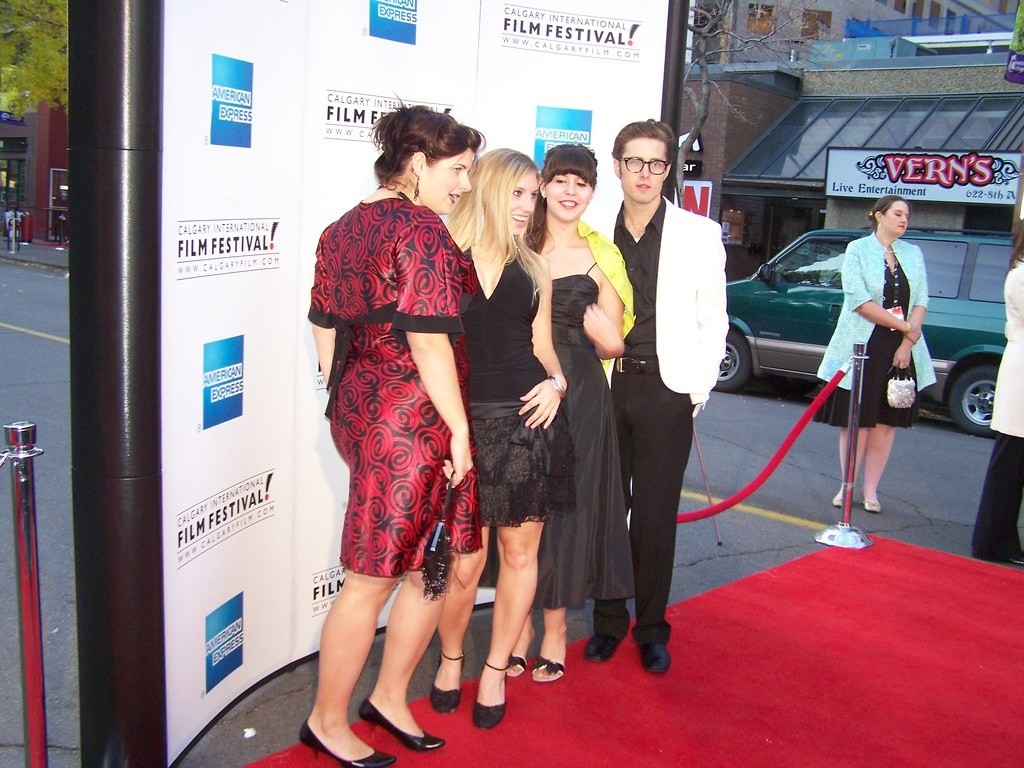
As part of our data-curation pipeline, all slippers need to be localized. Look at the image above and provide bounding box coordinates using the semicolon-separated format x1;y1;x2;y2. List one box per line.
531;655;565;682
506;655;527;677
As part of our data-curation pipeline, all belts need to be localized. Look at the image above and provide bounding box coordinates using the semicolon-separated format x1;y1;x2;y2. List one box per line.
614;357;659;373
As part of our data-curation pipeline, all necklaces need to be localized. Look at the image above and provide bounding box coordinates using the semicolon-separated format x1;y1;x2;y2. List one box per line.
886;251;894;254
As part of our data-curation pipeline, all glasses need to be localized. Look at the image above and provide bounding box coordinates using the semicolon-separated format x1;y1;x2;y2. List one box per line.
618;157;670;176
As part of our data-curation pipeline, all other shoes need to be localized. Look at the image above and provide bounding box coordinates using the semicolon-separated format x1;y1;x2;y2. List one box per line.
1008;551;1024;564
430;651;464;714
472;659;509;730
832;486;856;507
861;490;881;512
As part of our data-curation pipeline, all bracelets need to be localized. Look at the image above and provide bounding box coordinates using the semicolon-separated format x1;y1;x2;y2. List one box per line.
549;375;565;392
905;322;910;335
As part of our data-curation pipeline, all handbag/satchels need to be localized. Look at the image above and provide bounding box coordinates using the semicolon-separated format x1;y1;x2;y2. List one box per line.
885;365;916;409
421;469;459;602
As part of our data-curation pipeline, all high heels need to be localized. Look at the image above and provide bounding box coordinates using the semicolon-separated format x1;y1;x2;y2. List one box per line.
299;719;397;768
358;696;446;752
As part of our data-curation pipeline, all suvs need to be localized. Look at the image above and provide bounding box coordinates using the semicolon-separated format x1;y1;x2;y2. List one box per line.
712;225;1016;440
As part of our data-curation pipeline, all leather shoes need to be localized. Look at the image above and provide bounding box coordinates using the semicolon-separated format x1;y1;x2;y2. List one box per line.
584;635;620;663
639;642;669;672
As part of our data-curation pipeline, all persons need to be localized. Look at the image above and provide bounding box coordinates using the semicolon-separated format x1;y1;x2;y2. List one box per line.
477;143;637;682
431;149;567;730
810;196;938;513
299;105;479;768
583;120;728;674
968;214;1024;567
